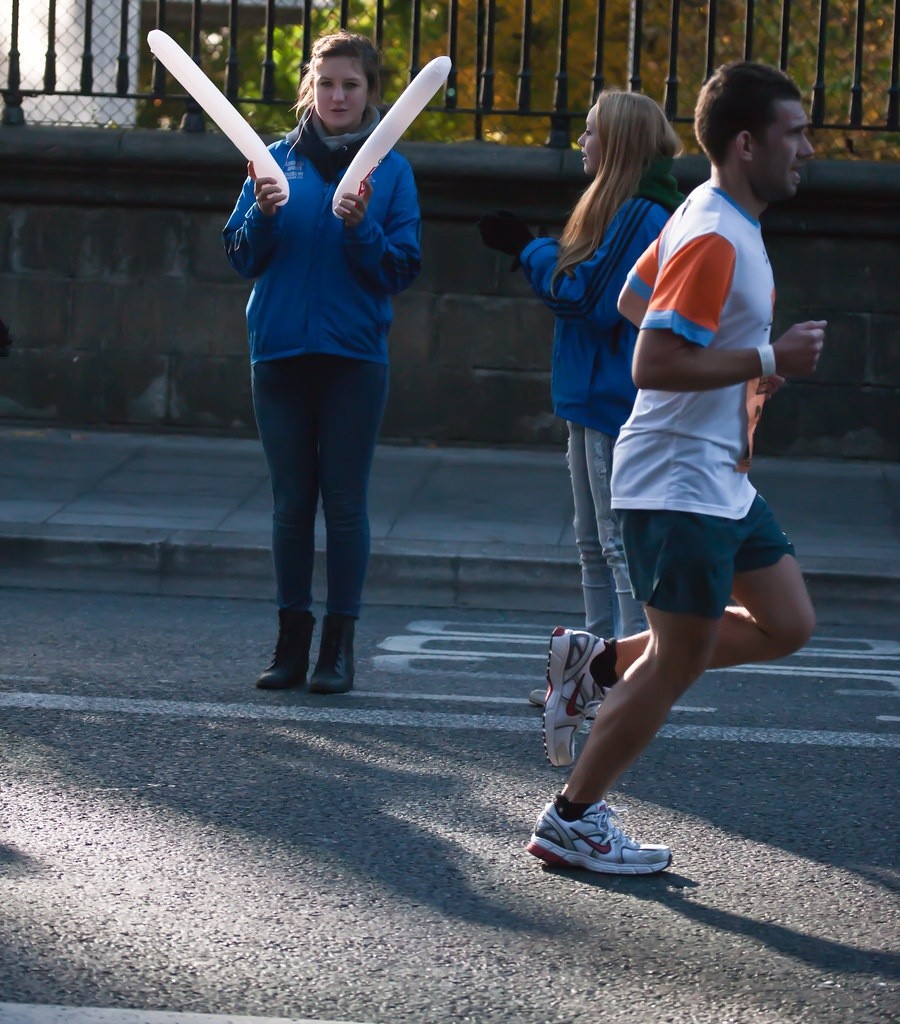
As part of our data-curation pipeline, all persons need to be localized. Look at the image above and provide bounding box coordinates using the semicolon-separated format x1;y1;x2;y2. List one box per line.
221;29;423;691
522;90;688;717
528;63;825;874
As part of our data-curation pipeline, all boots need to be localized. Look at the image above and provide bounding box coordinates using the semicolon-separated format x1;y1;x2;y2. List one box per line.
310;614;355;693
255;613;316;688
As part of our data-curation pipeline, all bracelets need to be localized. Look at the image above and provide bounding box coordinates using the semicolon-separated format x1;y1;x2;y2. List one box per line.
755;345;776;379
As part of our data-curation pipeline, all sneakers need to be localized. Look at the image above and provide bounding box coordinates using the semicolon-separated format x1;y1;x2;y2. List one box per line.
527;801;672;875
543;626;606;766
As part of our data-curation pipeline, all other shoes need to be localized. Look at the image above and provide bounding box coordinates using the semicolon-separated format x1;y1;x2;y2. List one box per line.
529;690;547;706
